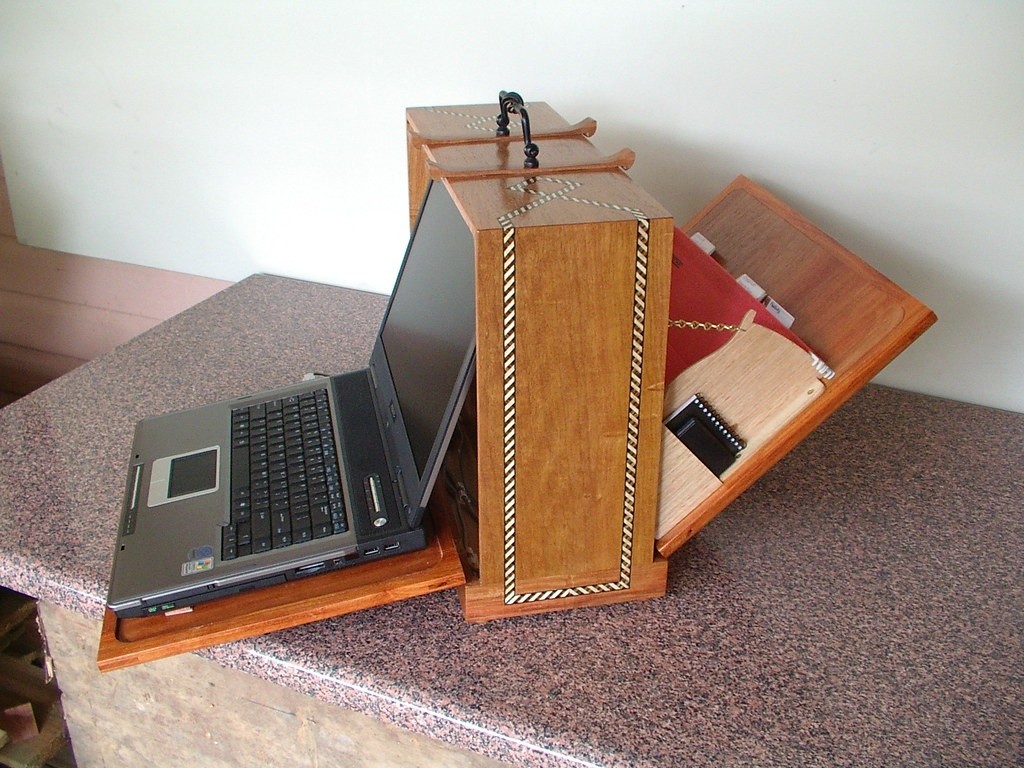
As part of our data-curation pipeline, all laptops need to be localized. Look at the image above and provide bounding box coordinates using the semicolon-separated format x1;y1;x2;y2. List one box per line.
108;178;478;620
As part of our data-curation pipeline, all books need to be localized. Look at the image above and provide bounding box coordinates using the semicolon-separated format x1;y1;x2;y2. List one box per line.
662;394;747;476
664;226;836;388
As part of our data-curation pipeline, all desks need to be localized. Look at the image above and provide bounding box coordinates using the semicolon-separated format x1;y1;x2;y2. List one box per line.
0;272;1024;768
